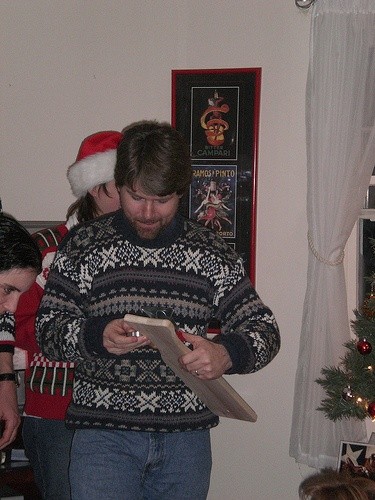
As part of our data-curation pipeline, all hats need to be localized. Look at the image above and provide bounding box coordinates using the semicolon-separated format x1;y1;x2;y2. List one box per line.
68;131;122;196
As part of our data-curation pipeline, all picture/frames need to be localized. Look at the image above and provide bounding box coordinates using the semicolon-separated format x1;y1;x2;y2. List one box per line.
170;67;263;288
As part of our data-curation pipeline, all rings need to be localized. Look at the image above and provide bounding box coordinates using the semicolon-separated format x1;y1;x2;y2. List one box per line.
193;369;199;377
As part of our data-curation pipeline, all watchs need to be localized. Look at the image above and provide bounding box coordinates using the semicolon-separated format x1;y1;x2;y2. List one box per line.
0;372;22;388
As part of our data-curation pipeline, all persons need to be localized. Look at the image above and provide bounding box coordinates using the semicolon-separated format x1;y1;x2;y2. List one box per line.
298;464;375;500
33;121;280;500
0;212;42;451
17;130;123;500
